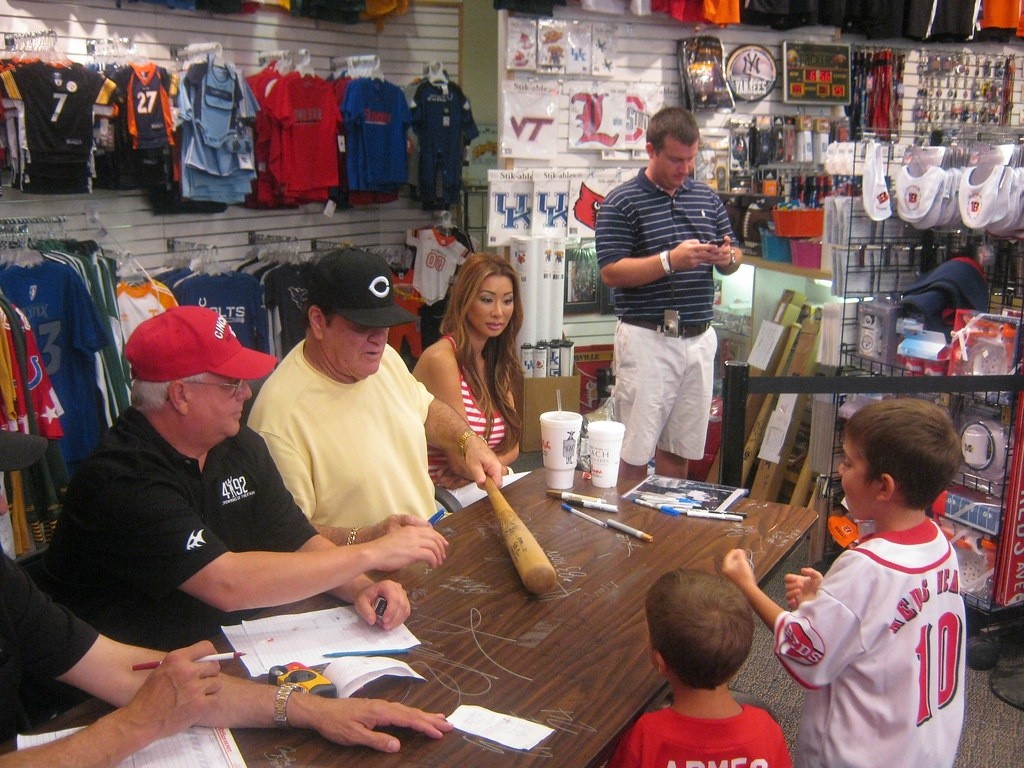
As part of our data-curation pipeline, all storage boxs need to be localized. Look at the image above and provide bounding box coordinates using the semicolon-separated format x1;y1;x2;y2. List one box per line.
510;331;580;453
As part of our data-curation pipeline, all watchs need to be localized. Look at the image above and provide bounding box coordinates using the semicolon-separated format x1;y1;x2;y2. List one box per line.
272;682;308;728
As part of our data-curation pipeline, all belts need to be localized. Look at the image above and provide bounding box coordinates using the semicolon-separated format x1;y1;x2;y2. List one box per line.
618;314;711;339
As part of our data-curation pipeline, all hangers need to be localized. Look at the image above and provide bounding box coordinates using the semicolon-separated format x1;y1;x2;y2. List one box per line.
87;37;150;68
251;233;304;267
2;30;69;64
170;41;236;72
308;239;408;276
0;214;147;287
164;239;224;276
428;210;457;238
328;55;386;84
420;60;450;85
245;48;318;80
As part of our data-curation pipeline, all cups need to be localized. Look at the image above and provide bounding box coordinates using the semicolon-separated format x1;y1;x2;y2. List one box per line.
539;410;583;491
587;420;625;488
508;234;566;365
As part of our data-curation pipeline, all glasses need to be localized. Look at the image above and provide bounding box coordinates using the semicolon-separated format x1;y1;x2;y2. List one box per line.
167;378;242;401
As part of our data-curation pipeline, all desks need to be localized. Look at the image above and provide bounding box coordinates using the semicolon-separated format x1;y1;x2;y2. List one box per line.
0;468;819;768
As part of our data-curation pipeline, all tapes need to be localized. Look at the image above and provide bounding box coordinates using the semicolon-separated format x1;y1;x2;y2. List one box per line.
961;420;1004;472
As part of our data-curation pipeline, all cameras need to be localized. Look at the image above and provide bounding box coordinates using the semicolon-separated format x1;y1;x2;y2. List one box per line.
664;309;680;338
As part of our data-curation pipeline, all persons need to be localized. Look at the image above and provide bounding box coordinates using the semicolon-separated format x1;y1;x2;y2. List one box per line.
411;251;525;512
721;397;967;768
595;111;743;480
0;428;454;768
604;568;792;768
246;247;504;548
32;305;449;653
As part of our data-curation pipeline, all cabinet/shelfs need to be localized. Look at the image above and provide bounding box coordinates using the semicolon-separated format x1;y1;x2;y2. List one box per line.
713;255;832;493
832;125;1024;622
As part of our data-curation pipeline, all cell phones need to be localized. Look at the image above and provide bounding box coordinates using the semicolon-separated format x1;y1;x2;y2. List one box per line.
707;238;726;248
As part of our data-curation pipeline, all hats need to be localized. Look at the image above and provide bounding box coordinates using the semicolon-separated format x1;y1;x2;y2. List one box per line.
0;428;48;470
126;305;279;382
305;247;419;327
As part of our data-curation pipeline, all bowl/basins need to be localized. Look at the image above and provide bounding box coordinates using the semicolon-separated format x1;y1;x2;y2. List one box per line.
761;231;791;261
772;205;823;239
789;238;820;269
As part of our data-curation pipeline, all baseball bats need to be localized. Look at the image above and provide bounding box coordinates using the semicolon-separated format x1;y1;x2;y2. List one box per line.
788;453;822;509
484;475;557;595
742;321;803;486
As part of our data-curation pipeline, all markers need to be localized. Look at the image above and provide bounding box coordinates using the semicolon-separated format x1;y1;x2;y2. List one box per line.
565;500;618;513
546;490;607;504
607;519;653;542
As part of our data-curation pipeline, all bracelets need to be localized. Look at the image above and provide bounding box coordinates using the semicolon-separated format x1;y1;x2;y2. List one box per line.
722;248;736;273
660;251;675;275
457;430;488;456
346;526;364;545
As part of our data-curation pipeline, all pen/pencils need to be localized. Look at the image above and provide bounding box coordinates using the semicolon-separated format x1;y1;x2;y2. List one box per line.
634;495;701;515
323;649;409;658
427;509;445;526
561;503;607;527
132;652;247;670
686;509;747;521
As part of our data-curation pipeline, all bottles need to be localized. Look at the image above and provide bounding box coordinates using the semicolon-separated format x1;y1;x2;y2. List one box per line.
783;169;852;206
521;338;574;378
